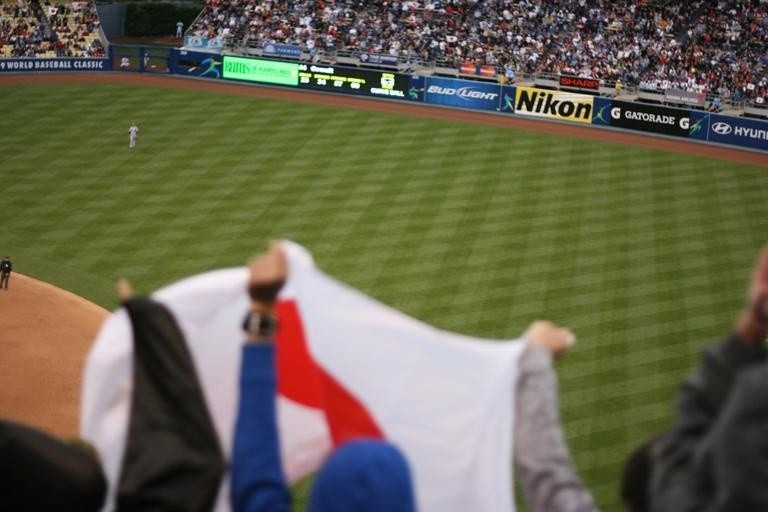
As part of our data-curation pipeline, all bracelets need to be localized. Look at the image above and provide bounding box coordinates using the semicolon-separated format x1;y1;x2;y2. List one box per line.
240;310;277;336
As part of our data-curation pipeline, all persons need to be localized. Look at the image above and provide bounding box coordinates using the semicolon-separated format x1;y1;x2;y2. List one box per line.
646;242;767;512
0;0;108;59
117;56;132;70
0;274;226;512
507;319;669;512
225;242;416;512
183;1;768;116
0;256;12;288
127;121;140;150
175;19;184;41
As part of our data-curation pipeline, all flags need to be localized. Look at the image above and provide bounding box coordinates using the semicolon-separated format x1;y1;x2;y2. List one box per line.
76;237;577;512
